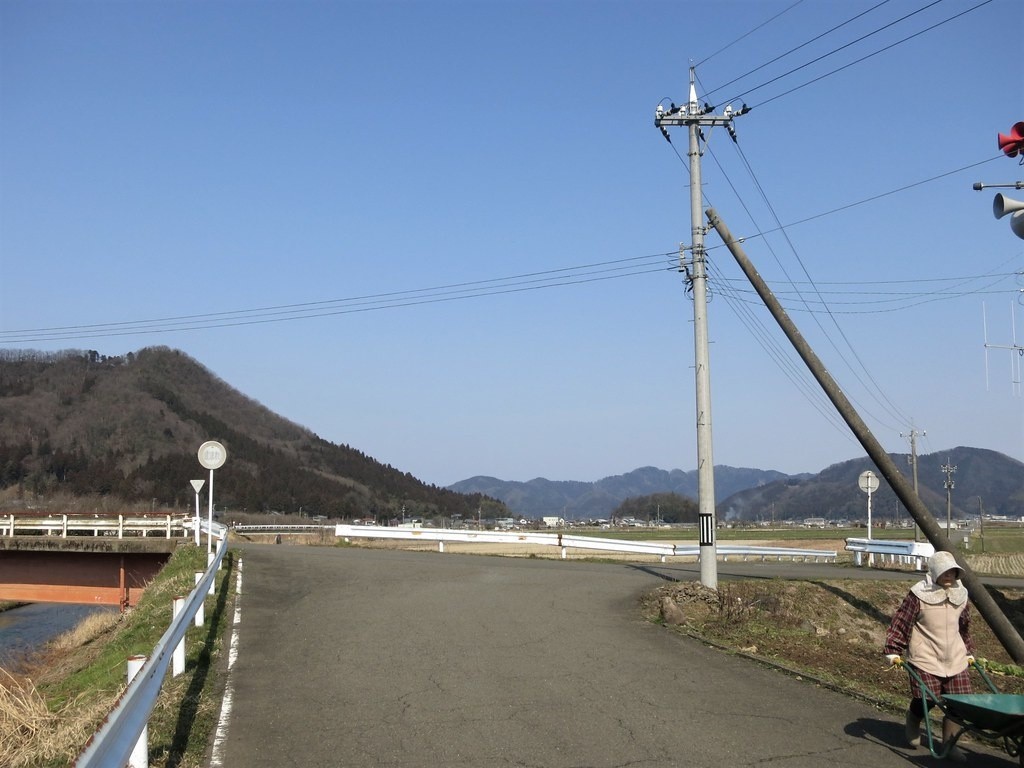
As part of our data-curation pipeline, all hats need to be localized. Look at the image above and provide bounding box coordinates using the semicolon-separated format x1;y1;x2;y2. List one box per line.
910;551;969;606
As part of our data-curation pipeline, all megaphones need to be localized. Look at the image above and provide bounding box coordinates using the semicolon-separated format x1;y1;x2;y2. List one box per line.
1010;209;1024;239
992;193;1024;220
997;121;1024;158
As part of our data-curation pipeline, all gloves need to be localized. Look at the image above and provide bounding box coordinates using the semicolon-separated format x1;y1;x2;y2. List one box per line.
882;653;901;667
966;654;976;666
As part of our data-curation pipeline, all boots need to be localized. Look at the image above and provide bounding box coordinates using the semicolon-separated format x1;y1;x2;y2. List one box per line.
904;711;922;747
939;716;968;765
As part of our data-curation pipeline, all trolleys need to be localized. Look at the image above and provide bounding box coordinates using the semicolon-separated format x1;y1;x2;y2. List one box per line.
895;658;1024;762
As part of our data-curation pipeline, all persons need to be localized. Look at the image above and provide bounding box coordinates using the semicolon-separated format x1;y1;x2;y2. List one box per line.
884;550;975;766
